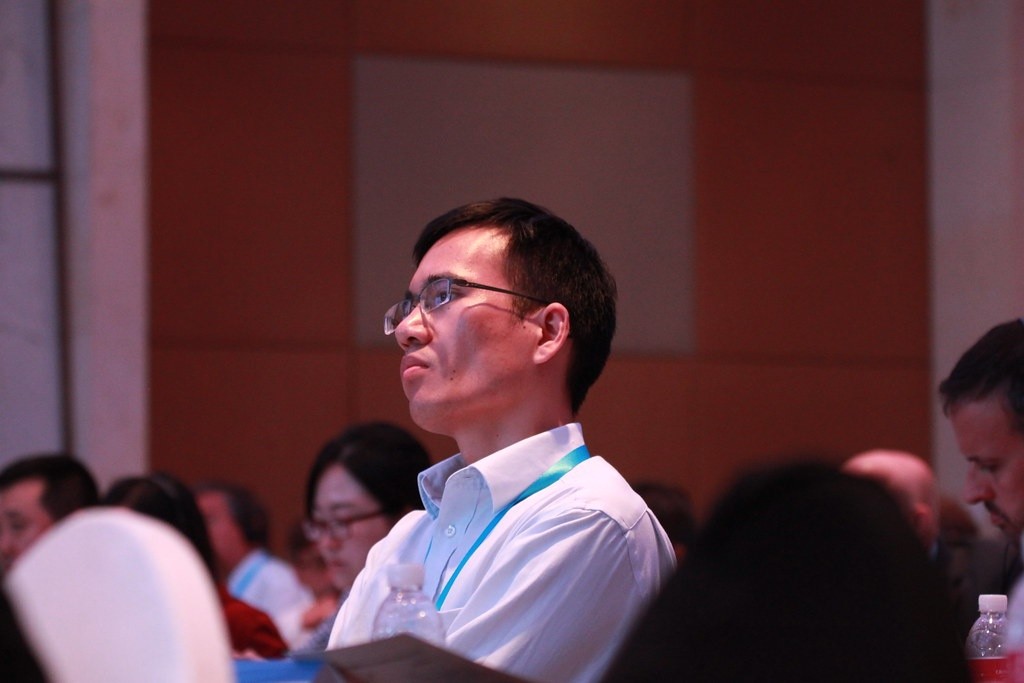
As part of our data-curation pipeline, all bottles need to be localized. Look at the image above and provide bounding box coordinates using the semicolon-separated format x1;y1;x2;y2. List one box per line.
964;594;1019;683
369;563;445;648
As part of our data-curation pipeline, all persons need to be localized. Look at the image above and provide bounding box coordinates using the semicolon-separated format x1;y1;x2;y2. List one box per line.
323;198;677;683
193;482;319;653
598;460;976;683
1;451;98;575
107;470;289;661
937;318;1024;683
294;422;434;655
840;449;941;550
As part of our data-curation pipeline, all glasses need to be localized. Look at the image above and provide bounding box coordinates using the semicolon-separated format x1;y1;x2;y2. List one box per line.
384;278;576;339
302;510;386;542
